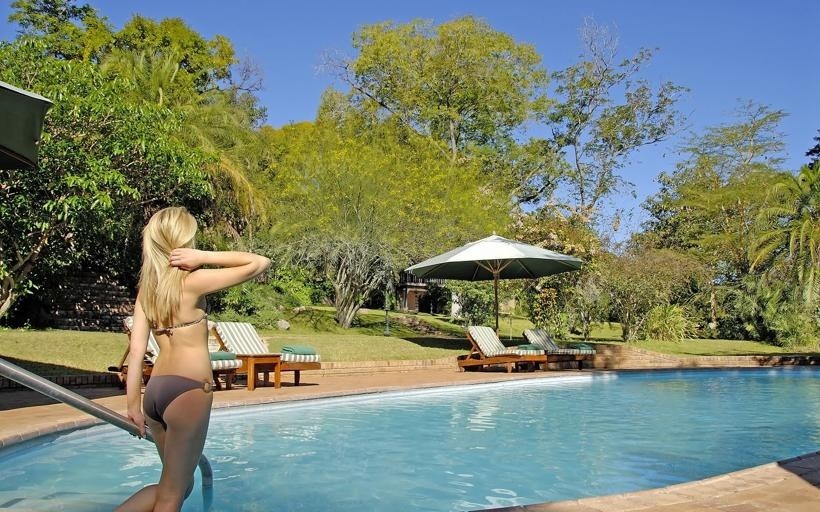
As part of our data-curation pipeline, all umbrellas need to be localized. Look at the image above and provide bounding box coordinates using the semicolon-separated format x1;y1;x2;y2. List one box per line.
403;233;583;339
0;80;55;174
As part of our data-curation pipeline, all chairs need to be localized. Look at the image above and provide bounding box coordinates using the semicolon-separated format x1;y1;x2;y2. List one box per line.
115;315;321;391
457;326;596;373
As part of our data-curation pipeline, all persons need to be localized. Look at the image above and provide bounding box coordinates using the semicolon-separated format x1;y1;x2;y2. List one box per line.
110;206;272;511
